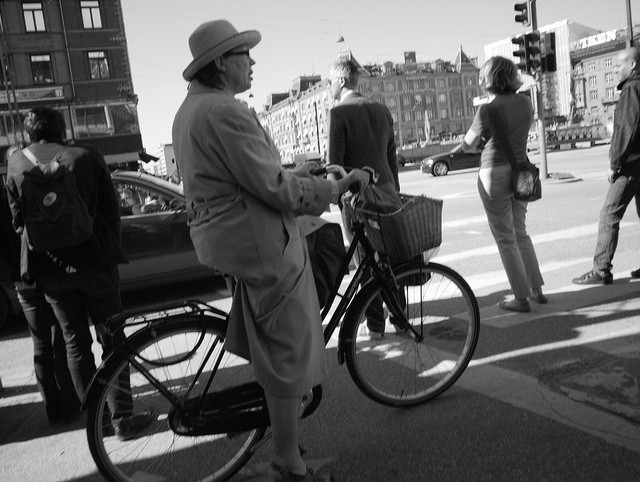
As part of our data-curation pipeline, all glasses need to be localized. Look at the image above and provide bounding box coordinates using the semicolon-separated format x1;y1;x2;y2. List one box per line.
224;47;250;57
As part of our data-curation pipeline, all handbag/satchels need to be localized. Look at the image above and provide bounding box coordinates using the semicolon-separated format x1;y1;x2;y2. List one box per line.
485;102;542;202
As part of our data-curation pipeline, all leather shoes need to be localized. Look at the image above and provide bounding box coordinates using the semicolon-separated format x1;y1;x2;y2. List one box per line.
528;292;547;304
631;268;640;277
499;301;530;312
572;269;613;284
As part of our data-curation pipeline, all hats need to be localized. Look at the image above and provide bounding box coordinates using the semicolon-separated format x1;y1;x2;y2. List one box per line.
182;19;261;81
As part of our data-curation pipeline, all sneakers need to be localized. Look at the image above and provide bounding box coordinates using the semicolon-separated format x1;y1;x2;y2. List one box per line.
115;409;155;441
381;317;405;334
355;322;382;340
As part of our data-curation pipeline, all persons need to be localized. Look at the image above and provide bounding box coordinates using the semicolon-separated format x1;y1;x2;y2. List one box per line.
5;130;77;422
465;54;546;311
570;41;639;284
171;22;373;482
329;61;411;338
119;190;150;214
13;110;139;437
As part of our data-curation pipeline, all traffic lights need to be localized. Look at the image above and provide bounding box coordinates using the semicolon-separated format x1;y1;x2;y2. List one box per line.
512;35;527;72
528;32;541;69
546;32;556;72
515;2;527;21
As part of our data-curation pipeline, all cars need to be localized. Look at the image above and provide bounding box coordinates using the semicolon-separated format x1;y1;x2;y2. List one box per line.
110;171;215;293
421;143;482;176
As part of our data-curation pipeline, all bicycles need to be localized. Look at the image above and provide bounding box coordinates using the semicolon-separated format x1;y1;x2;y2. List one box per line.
81;165;481;482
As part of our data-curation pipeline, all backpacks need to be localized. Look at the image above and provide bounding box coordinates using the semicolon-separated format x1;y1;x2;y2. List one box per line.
21;139;99;252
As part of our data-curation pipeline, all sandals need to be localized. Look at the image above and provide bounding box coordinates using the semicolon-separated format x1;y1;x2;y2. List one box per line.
270;460;334;482
